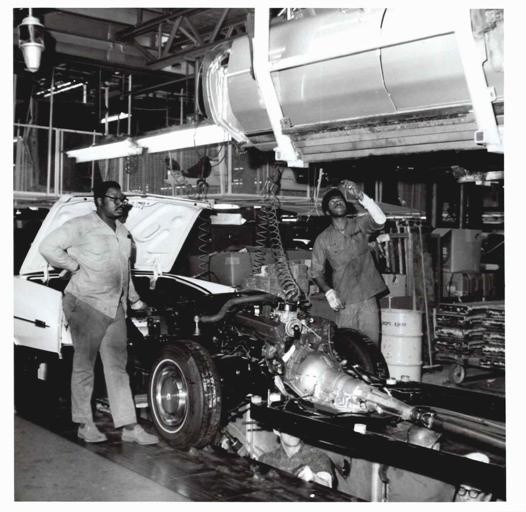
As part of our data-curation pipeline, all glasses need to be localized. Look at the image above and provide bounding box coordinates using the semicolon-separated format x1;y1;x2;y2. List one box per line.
102;194;128;205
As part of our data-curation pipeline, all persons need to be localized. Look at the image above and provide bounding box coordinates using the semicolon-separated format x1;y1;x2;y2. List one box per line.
307;180;391;360
37;180;162;448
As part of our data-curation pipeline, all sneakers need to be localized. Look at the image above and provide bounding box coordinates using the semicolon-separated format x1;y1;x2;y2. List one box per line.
78;422;107;442
123;426;160;446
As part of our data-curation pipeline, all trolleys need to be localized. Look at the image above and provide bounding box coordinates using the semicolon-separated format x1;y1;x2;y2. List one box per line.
436;271;506;385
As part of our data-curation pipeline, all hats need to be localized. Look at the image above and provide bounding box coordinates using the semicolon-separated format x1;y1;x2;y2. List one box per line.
322;188;342;212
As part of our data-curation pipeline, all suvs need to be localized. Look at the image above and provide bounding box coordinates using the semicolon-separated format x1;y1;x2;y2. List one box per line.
13;192;390;452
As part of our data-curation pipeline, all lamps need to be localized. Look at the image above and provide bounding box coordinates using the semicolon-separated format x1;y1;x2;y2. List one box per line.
16;8;45;72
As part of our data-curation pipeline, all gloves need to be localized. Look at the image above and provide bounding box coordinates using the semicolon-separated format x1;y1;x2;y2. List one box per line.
326;290;344;312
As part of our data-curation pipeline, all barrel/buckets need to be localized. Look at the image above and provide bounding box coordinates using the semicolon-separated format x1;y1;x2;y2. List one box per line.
380;307;424;382
